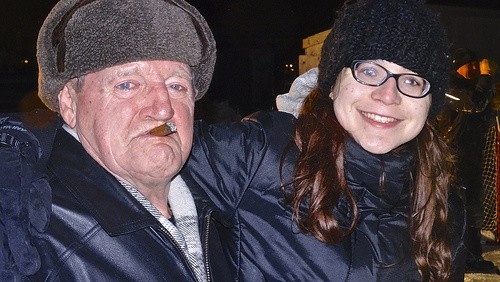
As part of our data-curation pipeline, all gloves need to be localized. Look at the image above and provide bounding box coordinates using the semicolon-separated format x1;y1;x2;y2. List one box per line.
0;121;54;282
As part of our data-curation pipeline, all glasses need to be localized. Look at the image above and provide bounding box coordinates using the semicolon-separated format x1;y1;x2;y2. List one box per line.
346;59;438;99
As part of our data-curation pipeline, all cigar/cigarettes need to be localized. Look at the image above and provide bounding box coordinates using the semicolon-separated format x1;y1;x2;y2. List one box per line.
148;123;176;138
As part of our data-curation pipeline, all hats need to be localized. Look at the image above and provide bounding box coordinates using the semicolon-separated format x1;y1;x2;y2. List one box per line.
37;0;217;112
454;48;478;70
316;0;452;98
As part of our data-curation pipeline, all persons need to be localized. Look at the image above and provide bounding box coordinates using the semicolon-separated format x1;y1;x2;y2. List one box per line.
0;0;318;282
453;46;500;274
0;0;476;282
